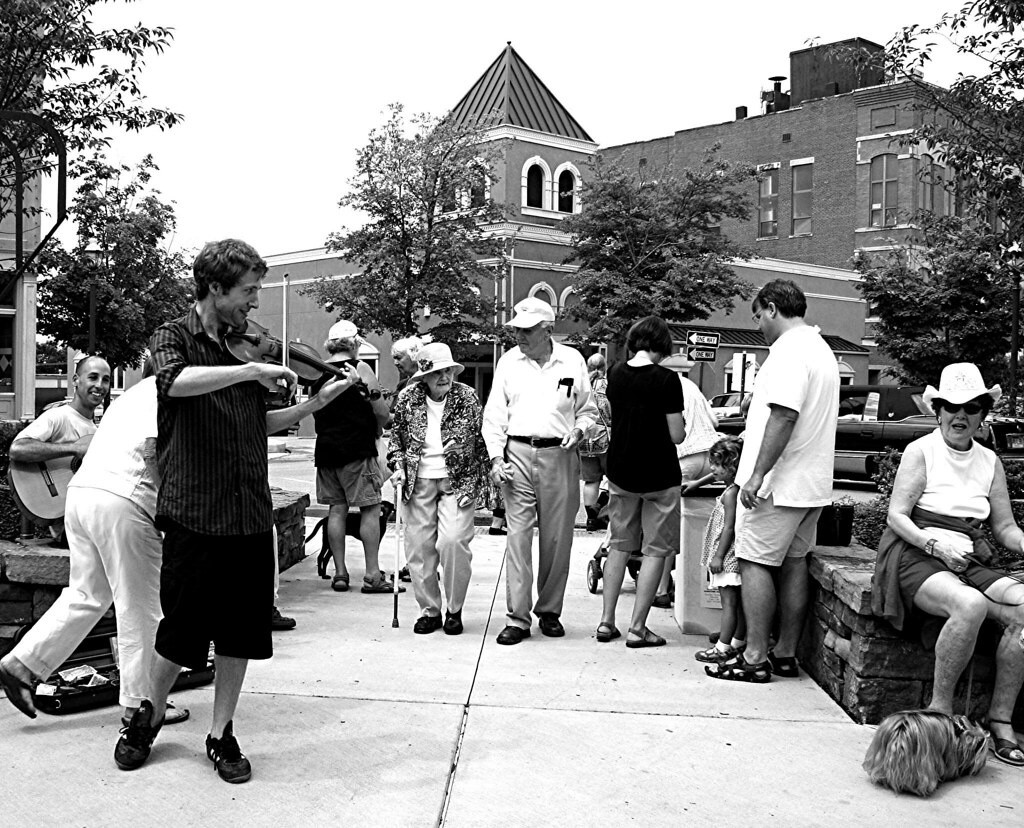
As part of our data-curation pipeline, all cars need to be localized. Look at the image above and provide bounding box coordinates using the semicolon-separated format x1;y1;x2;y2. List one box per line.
833;383;1024;495
708;393;749;427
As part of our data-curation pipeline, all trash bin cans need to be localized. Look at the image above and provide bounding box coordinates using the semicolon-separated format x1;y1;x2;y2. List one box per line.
673;480;728;635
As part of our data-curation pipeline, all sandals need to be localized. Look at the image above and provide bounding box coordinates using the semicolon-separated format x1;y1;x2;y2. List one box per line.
625;625;667;648
705;650;799;682
596;622;621;642
331;573;349;592
399;563;441;582
361;570;406;594
695;643;748;663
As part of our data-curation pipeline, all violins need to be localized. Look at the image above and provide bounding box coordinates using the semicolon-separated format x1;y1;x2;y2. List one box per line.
220;318;382;401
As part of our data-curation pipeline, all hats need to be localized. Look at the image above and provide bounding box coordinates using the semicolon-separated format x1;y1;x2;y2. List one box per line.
923;362;1002;416
328;320;366;344
410;342;465;381
505;297;555;329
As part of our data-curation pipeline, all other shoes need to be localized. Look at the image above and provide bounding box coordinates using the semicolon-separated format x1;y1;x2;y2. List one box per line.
650;589;674;609
586;518;607;531
272;609;297;629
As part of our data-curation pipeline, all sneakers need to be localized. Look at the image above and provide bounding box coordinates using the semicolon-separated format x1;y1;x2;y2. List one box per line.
113;699;167;770
208;719;252;784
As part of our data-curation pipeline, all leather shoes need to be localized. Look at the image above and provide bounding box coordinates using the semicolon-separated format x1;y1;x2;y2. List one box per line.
414;609;463;635
496;615;565;645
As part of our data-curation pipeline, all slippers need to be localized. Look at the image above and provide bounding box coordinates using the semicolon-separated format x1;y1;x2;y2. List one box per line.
120;703;190;727
0;663;38;719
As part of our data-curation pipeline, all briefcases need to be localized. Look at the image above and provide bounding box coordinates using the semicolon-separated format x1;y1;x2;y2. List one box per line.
11;617;216;717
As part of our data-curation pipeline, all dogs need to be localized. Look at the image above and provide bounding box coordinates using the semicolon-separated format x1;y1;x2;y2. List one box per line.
863;708;995;796
305;501;394;580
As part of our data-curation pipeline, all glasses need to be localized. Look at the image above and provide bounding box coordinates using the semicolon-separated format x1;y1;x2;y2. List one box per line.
941;402;983;415
752;308;764;324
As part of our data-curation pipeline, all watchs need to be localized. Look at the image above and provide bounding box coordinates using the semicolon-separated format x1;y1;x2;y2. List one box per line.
925;537;939;558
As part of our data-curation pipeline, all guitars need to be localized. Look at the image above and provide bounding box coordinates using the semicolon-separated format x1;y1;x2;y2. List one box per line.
7;441;84;527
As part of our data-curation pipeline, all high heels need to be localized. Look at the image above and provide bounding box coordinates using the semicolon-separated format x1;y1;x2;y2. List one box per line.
980;711;1024;766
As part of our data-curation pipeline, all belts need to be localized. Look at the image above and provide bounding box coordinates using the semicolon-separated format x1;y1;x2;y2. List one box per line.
507;435;570;449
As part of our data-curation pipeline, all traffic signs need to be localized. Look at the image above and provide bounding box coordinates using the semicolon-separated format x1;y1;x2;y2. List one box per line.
685;330;720;349
686;346;717;363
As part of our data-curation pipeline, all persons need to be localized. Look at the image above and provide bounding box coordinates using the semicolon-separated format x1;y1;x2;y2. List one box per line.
595;316;687;648
115;240;361;783
871;362;1024;767
577;353;613;531
704;279;839;684
308;319;407;594
681;392;753;664
0;355;297;726
650;376;721;609
383;297;600;646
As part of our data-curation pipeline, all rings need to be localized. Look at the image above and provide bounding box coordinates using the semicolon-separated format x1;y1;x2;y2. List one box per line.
745;497;749;500
955;565;962;572
